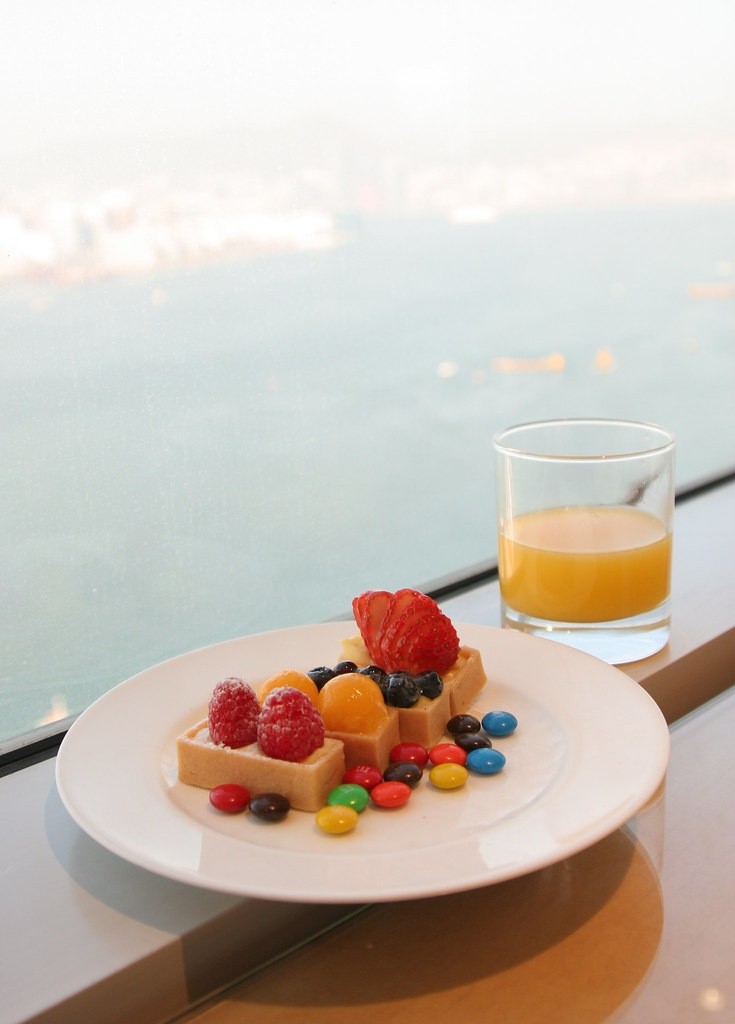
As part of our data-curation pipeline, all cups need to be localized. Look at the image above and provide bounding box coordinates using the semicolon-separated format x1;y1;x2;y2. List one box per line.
492;418;676;665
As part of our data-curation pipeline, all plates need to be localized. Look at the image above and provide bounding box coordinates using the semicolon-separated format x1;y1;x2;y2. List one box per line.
52;616;670;904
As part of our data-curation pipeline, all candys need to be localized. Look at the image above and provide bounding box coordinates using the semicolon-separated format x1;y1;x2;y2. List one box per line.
208;711;517;834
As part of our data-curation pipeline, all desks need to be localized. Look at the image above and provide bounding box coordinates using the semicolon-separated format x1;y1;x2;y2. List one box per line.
0;475;735;1024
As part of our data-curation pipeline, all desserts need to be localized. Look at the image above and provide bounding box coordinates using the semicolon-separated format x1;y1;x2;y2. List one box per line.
177;589;488;811
